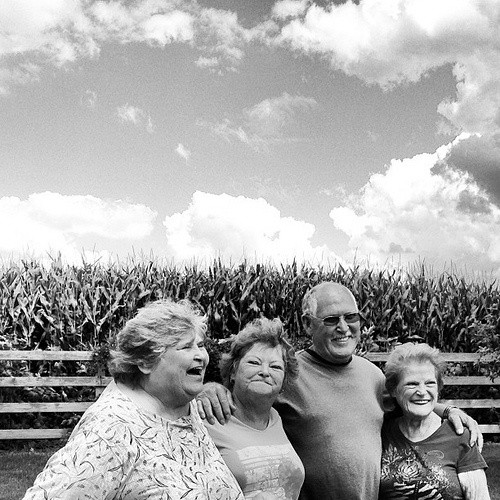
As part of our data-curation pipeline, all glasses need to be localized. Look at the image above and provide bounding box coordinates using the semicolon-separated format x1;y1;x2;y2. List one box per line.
309;313;359;326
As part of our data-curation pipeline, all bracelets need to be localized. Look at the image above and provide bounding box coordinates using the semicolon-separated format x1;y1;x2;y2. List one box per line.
443;405;458;419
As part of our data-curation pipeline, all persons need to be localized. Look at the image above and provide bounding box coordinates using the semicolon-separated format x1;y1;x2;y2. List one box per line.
195;281;484;500
202;316;305;500
379;343;490;500
20;298;245;500
